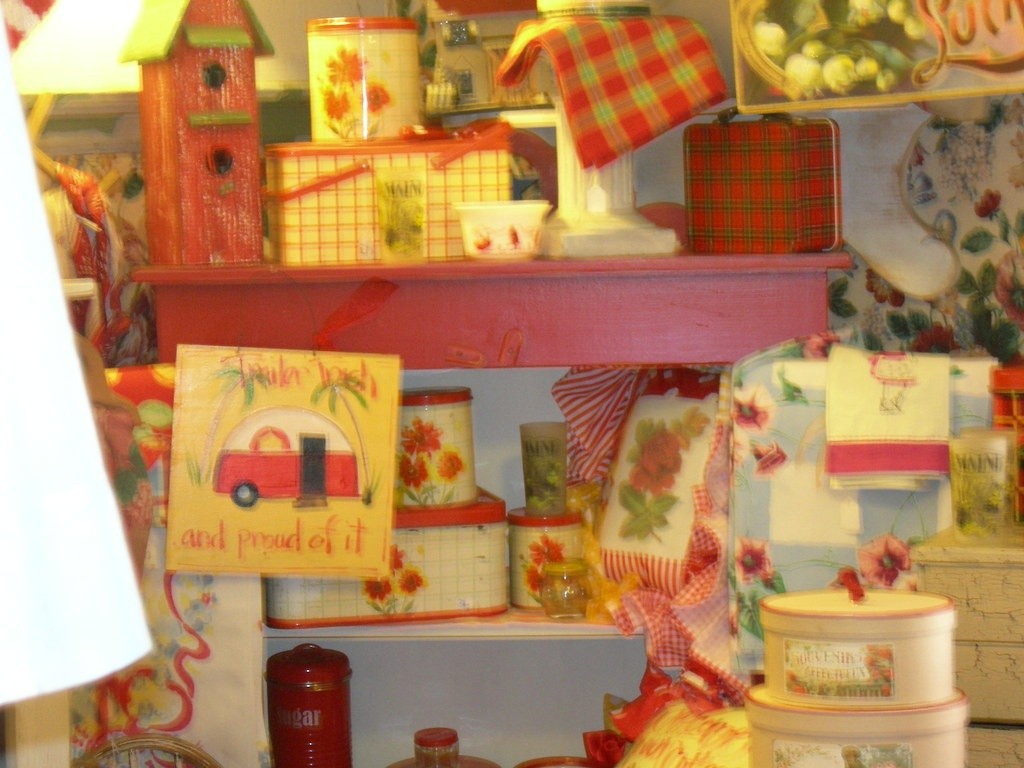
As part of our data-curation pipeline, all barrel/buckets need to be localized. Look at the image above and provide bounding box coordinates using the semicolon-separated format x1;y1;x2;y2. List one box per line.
393;382;479;507
745;589;967;768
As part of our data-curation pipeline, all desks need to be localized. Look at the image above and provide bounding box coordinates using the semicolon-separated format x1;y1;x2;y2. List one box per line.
131;246;857;375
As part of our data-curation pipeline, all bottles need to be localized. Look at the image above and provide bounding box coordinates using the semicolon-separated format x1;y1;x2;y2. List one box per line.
508;507;584;609
988;367;1024;522
266;643;353;768
384;728;501;768
309;17;423;141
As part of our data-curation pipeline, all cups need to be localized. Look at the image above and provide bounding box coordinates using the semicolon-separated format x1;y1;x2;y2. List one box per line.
948;427;1017;543
527;558;589;619
455;201;553;260
519;422;567;515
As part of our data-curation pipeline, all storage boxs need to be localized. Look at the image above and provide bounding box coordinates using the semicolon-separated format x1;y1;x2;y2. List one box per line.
744;681;971;768
759;587;958;706
